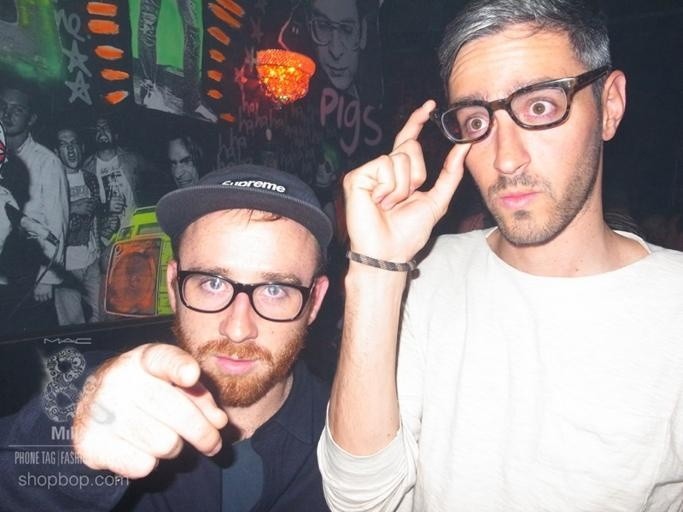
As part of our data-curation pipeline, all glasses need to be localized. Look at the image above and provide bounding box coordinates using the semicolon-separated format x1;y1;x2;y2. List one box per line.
310;17;360;49
430;65;609;144
174;268;315;321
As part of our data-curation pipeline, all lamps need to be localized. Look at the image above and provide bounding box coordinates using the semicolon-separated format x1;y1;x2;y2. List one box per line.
255;49;317;104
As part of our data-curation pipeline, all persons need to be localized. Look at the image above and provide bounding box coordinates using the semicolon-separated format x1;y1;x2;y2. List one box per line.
50;127;122;327
315;0;683;511
81;111;146;324
166;128;216;186
137;0;218;125
0;164;333;510
0;84;70;330
306;1;369;93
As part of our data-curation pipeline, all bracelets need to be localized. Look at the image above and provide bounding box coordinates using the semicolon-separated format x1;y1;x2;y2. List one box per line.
344;250;420;274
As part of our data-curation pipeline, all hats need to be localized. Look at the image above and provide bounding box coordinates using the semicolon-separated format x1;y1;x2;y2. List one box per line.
153;163;334;250
